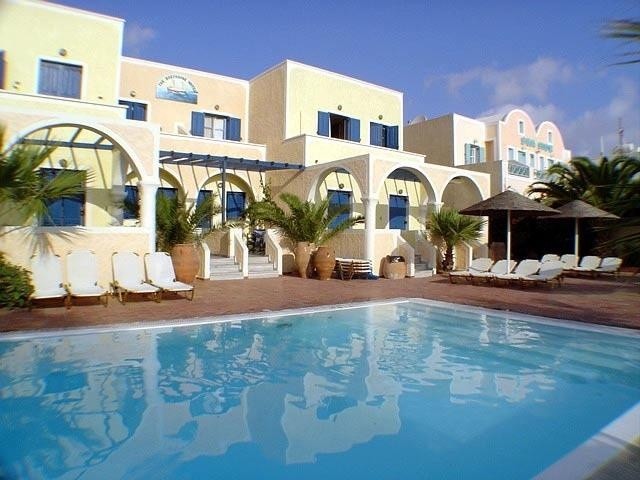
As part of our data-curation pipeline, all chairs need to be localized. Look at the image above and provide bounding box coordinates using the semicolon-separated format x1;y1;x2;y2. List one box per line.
109;251;160;304
145;252;195;301
335;256;372;282
541;254;623;281
67;249;109;307
450;258;565;291
28;255;69;310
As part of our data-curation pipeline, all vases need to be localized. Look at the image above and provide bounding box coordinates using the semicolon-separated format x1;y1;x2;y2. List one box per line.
314;247;337;280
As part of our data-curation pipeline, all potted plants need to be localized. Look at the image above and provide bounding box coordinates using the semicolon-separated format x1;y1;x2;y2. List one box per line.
242;190;367;278
156;187;248;285
427;205;488;276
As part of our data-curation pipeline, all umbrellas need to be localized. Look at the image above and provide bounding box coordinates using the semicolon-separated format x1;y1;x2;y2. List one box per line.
459;186;561;275
537;199;621;256
